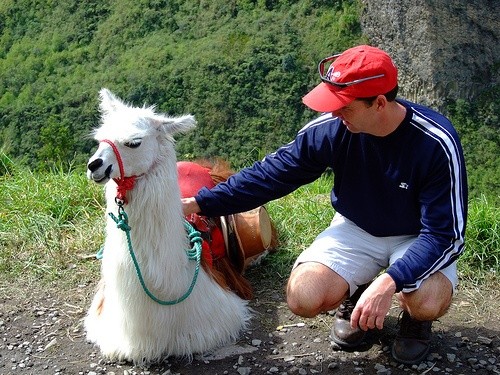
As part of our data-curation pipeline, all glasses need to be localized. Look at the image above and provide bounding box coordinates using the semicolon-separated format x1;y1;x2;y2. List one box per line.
318;53;386;92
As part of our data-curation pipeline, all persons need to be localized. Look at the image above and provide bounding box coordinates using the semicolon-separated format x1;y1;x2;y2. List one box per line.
182;45;469;362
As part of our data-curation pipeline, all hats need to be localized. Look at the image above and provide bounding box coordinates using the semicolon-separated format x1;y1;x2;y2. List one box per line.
302;45;398;112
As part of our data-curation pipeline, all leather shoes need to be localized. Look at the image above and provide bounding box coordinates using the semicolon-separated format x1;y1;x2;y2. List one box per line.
329;280;367;347
392;308;433;364
